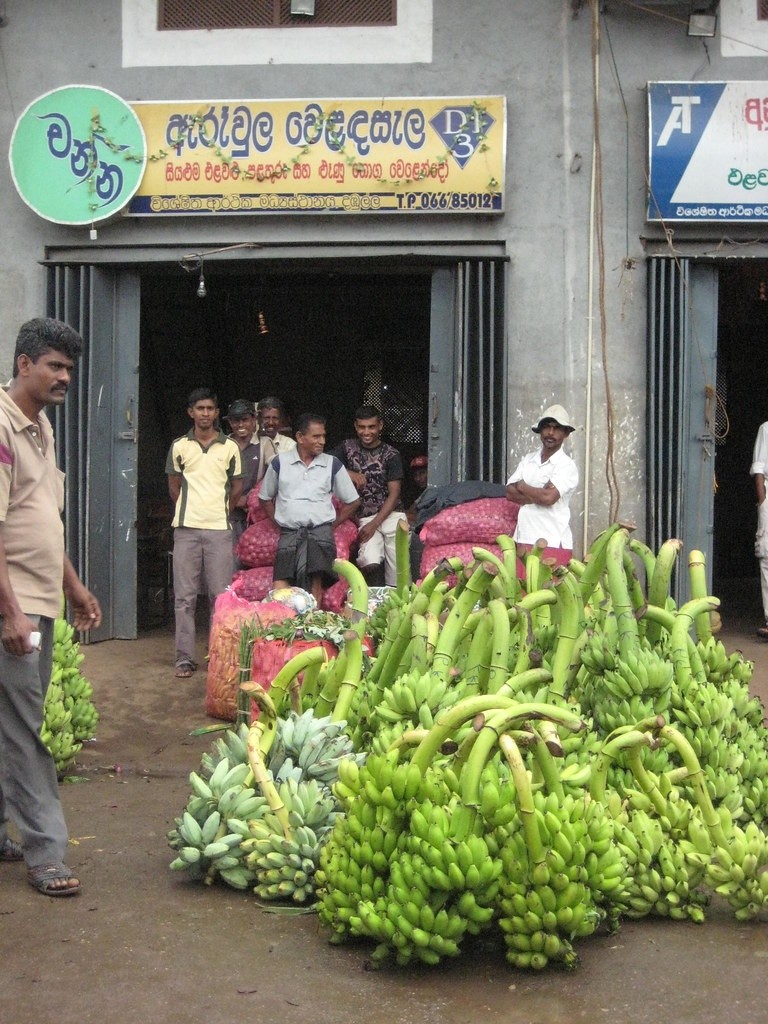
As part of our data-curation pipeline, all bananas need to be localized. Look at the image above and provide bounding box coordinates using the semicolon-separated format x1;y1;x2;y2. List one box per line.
39;622;99;770
165;708;368;902
275;600;768;970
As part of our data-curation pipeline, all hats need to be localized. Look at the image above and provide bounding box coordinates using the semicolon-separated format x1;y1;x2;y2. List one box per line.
532;404;575;434
222;399;255;419
410;454;428;468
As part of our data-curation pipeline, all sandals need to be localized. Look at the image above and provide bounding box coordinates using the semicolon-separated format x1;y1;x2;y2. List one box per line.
174;664;193;677
28;862;81;896
0;837;26;861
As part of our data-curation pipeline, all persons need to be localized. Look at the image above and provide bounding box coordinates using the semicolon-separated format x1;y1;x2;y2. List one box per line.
0;318;101;894
750;422;768;638
227;399;278;573
332;406;407;588
258;397;297;453
257;414;361;609
506;404;579;597
165;388;248;677
396;457;427;528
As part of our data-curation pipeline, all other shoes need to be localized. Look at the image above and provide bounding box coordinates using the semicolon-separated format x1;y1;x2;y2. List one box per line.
757;628;768;638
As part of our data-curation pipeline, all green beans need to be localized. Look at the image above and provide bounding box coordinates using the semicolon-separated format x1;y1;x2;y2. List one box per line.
204;607;288;720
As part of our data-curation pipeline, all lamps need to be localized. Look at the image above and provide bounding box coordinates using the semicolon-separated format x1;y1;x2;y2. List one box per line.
686;8;718;38
256;309;271;337
290;0;316;21
